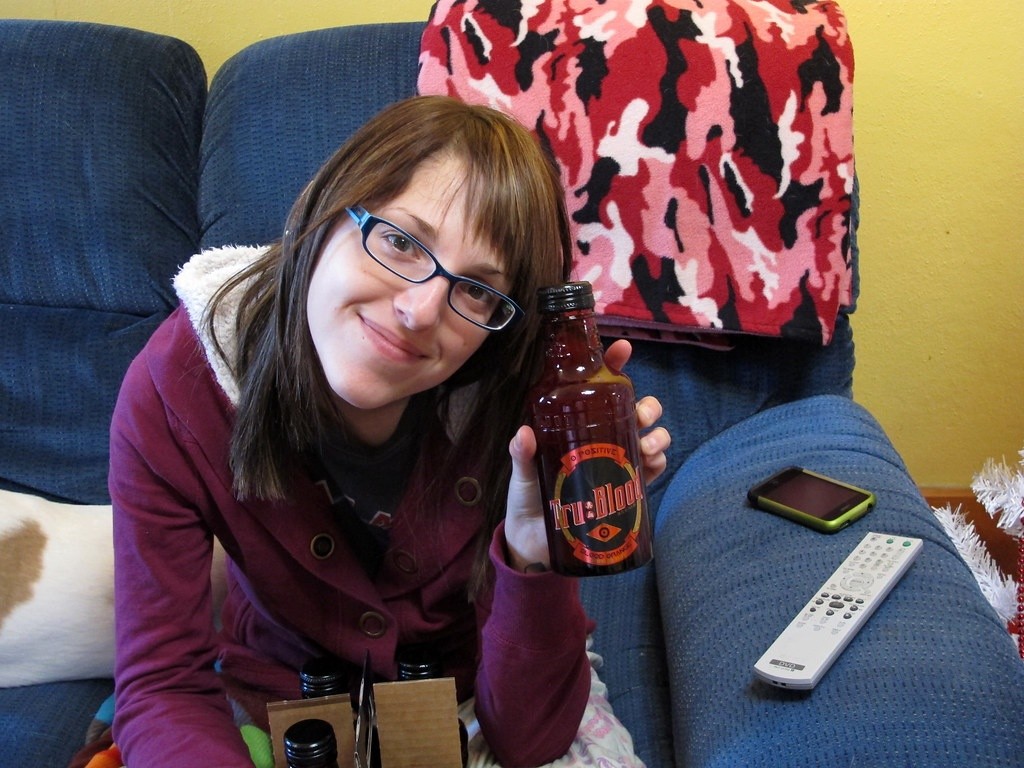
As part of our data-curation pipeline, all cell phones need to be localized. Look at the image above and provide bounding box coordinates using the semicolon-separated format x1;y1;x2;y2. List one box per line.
749;465;876;534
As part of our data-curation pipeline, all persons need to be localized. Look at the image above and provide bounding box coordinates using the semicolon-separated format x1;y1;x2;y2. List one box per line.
69;94;670;768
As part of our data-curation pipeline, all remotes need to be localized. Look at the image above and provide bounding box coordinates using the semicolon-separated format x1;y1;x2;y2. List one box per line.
752;533;926;690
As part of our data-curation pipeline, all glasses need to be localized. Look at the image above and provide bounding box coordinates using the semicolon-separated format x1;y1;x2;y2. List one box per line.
346;199;525;334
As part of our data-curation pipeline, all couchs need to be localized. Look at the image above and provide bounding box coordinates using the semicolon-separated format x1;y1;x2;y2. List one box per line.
0;17;1024;768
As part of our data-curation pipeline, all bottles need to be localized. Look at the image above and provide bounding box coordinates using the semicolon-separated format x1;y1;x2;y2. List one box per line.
299;664;338;699
284;719;339;768
397;655;433;680
528;280;652;577
